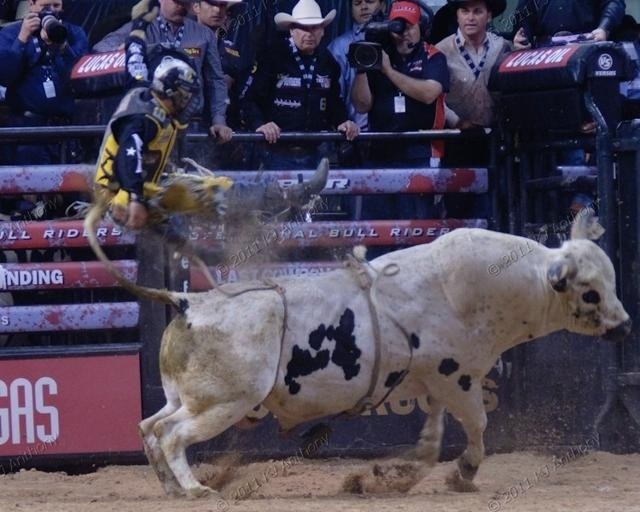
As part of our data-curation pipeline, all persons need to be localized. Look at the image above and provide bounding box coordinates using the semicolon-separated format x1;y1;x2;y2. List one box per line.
0;0;638;263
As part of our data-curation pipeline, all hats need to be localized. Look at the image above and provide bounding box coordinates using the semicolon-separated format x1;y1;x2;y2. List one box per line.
389;3;420;25
448;0;506;18
274;0;336;28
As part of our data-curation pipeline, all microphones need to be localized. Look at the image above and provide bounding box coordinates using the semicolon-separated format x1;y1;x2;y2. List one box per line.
408;36;426;48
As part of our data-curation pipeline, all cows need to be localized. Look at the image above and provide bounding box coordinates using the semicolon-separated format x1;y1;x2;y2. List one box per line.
84;186;632;502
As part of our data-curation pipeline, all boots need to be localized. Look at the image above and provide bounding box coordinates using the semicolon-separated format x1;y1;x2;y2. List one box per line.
286;157;330;207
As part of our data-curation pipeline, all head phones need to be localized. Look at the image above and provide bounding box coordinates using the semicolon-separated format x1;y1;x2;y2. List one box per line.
388;0;433;37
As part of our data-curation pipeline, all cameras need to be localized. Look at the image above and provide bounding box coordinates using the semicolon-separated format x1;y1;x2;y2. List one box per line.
32;7;67;41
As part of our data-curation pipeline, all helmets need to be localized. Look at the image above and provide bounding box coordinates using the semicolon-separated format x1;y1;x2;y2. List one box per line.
153;56;201;119
